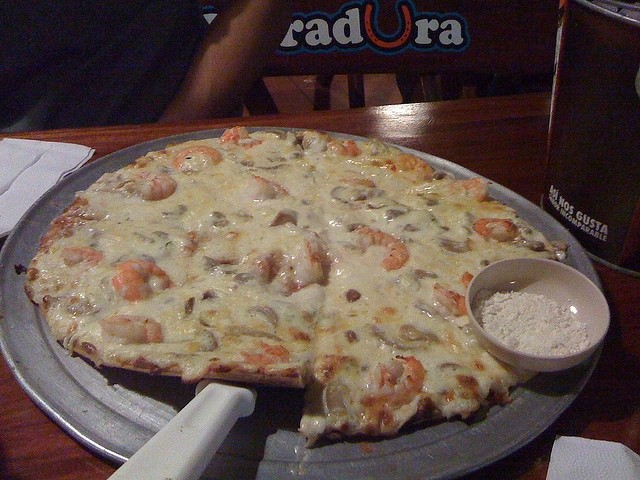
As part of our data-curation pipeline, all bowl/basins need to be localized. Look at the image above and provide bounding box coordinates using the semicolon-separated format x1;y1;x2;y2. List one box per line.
466;256;612;375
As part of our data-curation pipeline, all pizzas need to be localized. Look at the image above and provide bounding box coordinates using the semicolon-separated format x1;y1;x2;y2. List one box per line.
24;127;570;450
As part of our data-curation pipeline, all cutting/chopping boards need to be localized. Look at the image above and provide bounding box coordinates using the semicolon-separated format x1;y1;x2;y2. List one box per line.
1;125;606;479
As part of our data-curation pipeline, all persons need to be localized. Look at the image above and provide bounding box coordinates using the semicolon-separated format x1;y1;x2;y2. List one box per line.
1;0;298;132
